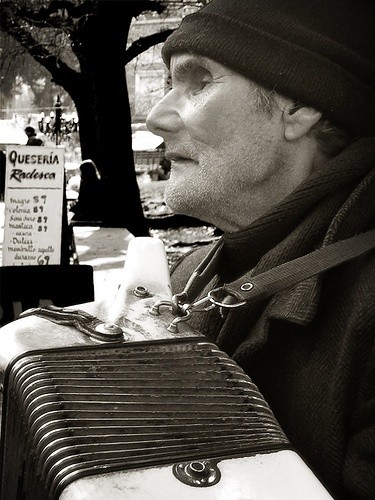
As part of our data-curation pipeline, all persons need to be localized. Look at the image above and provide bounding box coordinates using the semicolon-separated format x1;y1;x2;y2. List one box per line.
148;1;374;500
68;160;104;248
25;126;44;147
38;113;79;146
147;149;172;181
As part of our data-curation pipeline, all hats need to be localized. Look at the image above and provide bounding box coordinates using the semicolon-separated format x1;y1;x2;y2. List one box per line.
161;0;375;135
